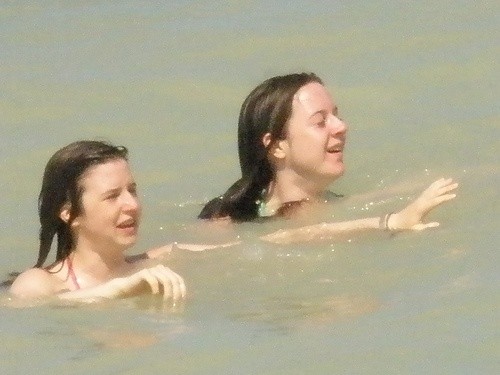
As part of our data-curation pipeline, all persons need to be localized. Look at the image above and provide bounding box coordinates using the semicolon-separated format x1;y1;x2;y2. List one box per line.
198;73;347;224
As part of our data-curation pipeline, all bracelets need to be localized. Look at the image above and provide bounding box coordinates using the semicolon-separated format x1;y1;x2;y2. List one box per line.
380;212;396;231
0;141;458;302
